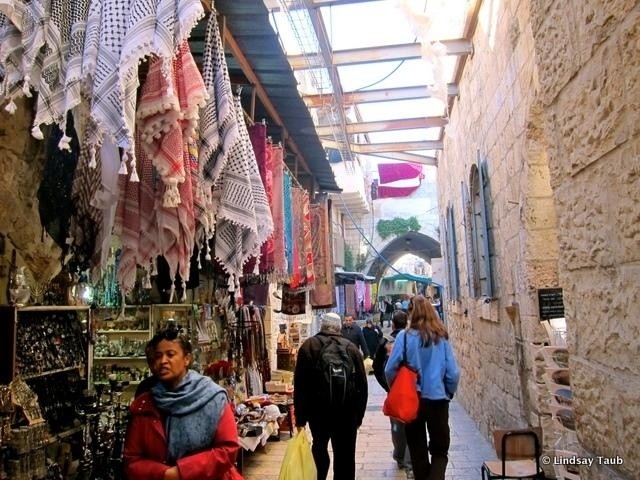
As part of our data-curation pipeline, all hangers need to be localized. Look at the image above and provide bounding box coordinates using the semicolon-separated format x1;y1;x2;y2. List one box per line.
208;0;324;207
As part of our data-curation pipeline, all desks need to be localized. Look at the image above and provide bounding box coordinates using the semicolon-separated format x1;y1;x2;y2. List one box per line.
278;350;290;368
237;368;296;474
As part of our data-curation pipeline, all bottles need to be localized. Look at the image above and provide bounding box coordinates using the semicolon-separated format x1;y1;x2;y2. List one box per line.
104;246;120;307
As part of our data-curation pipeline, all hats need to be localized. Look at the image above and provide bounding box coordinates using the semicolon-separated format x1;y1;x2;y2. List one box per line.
323;312;341;327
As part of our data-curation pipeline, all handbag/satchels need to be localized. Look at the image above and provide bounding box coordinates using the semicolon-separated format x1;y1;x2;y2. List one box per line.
278;427;318;480
382;366;421;424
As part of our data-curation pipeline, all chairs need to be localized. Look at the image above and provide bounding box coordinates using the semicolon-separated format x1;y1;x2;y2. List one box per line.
481;428;544;479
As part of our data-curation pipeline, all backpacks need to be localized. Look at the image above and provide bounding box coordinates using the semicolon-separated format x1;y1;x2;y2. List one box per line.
383;335;396;390
314;335;354;408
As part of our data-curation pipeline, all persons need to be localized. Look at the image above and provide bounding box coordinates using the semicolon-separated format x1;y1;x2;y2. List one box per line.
363;297;443;359
135;339;158;398
341;314;369;360
123;329;245;480
384;296;461;480
372;310;415;478
293;312;368;480
